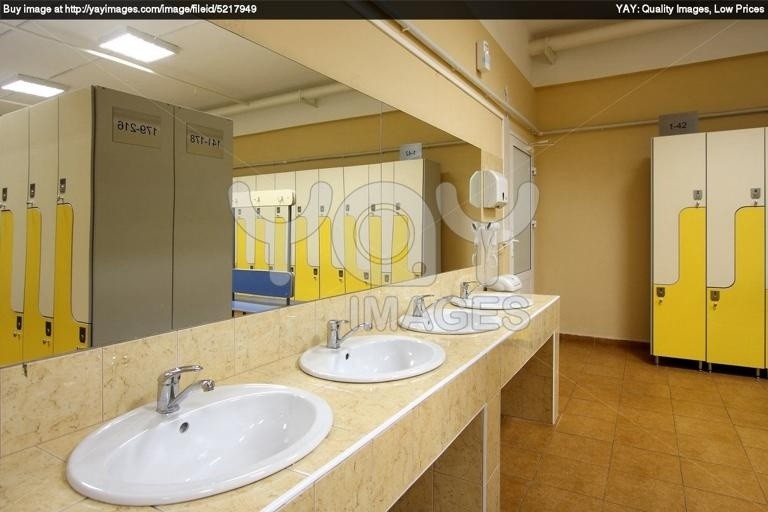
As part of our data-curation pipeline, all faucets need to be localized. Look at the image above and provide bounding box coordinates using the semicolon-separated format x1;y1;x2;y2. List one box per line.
460;281;488;298
326;319;373;349
413;294;454;317
155;364;215;414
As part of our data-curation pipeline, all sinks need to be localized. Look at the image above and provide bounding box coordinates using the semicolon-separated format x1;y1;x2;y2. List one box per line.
65;383;334;508
450;292;535;310
295;335;447;383
398;308;504;334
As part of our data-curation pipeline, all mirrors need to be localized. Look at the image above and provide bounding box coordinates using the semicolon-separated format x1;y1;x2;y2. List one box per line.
0;19;481;371
